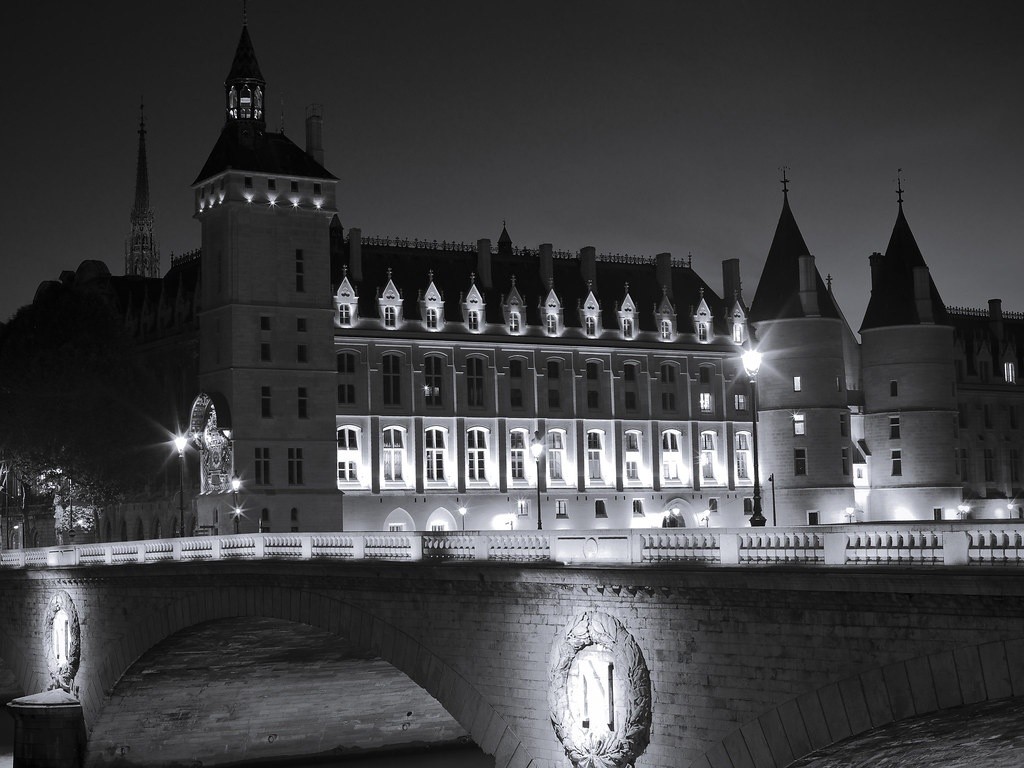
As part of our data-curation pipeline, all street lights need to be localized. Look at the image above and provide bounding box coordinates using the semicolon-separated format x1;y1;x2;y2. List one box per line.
531;443;543;530
742;348;767;526
173;436;188;539
